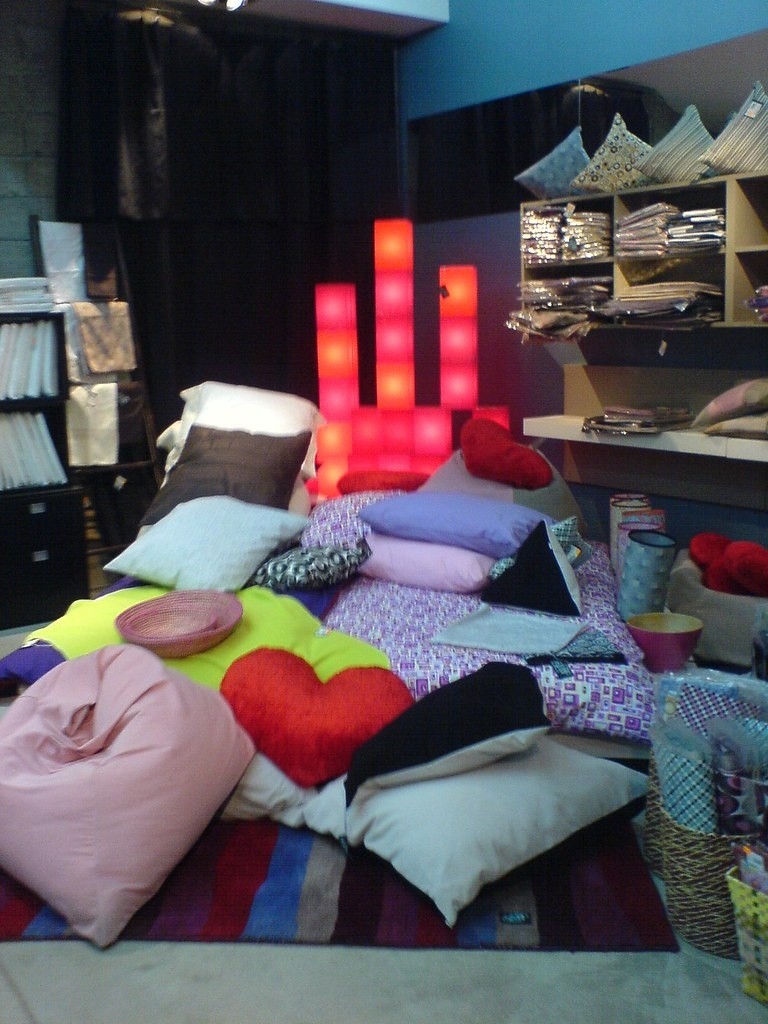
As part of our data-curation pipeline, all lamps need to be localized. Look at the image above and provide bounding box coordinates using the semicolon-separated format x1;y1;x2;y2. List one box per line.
303;216;511;500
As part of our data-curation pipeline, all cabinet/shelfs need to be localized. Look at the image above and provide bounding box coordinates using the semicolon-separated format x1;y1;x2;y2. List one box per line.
0;301;159;631
520;171;768;334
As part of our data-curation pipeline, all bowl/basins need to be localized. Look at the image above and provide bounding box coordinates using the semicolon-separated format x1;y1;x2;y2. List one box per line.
626;612;704;673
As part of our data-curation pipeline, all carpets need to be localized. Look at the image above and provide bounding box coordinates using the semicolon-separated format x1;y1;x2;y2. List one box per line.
0;822;680;954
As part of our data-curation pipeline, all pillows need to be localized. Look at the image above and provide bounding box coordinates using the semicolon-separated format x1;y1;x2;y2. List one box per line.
567;112;652;192
0;381;664;950
512;124;591;200
696;79;768;175
631;105;716;184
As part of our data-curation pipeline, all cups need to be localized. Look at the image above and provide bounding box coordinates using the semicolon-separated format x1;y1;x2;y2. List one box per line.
618;531;677;622
610;492;665;579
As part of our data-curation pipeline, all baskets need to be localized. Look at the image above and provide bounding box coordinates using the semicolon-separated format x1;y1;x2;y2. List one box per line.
643;748;663;880
117;589;242;658
726;865;768;1006
660;805;761;961
